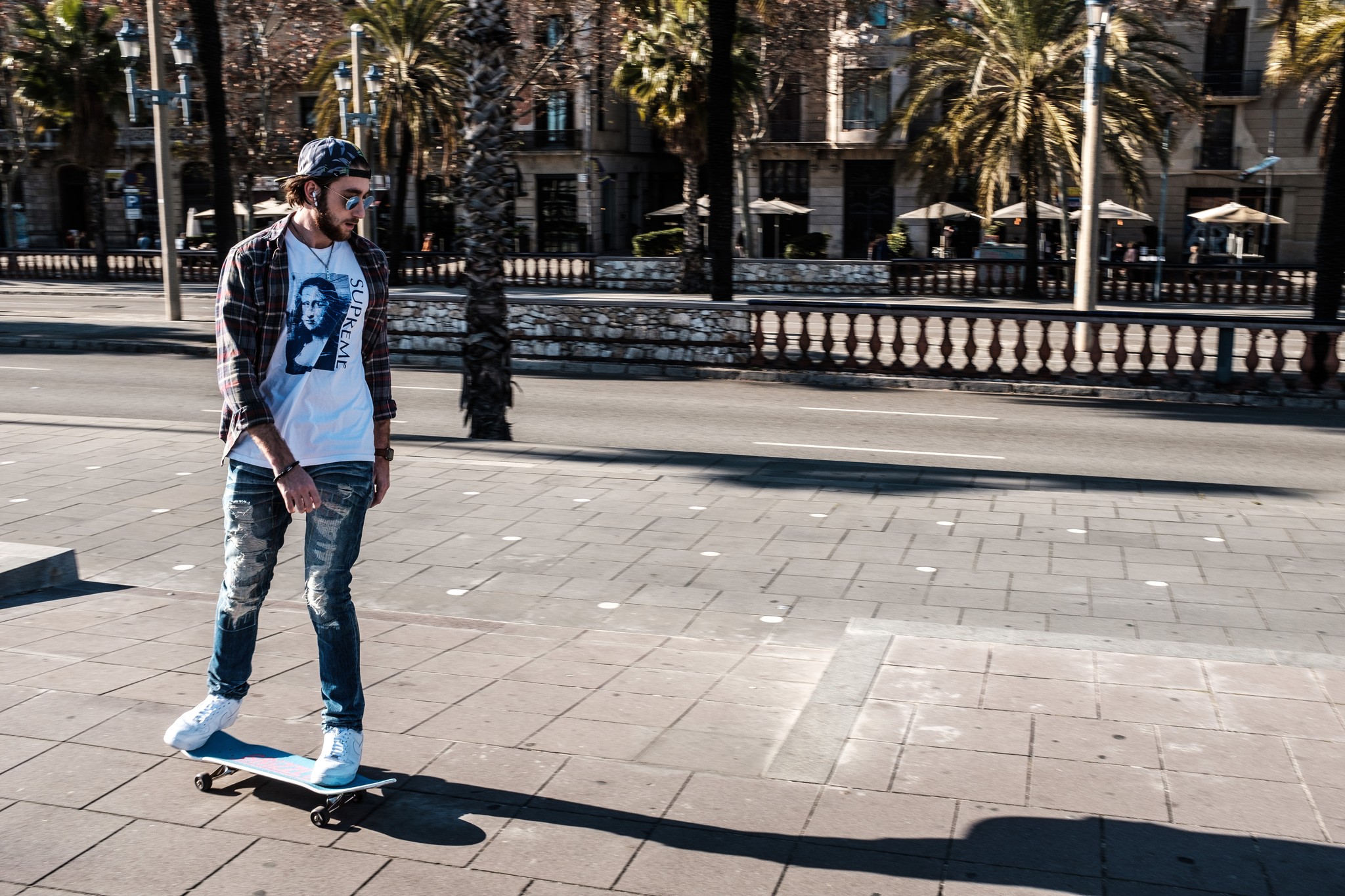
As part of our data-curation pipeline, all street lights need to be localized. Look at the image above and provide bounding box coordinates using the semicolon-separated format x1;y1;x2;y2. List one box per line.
116;1;194;321
1075;0;1119;356
335;23;384;245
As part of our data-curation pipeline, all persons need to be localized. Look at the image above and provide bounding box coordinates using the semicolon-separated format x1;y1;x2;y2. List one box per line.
162;135;391;794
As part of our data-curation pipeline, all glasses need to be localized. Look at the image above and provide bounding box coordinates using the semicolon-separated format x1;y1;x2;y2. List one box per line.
323;185;375;210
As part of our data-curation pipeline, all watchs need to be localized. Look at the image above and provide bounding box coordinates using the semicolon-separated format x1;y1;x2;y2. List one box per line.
375;447;394;461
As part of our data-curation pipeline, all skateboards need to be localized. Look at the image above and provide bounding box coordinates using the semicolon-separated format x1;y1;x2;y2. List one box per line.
180;730;397;827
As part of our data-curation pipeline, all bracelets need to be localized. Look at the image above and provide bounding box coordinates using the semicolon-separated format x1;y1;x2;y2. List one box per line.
274;460;300;483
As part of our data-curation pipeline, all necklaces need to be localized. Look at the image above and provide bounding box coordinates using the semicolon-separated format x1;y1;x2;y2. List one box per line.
288;215;337;281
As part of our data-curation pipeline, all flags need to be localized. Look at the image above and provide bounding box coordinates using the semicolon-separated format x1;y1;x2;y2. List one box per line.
1238;155;1282;177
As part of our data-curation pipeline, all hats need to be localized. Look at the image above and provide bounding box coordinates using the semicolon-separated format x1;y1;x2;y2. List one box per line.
273;136;371;183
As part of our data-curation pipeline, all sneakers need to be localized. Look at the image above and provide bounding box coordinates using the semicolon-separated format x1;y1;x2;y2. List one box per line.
310;727;363;786
163;693;242;751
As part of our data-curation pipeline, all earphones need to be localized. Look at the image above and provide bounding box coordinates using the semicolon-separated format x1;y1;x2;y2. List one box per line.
312;191;318;206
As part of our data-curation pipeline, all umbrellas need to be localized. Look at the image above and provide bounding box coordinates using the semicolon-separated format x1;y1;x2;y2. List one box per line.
191;197;301;238
1186;202;1290;234
894;200;986;234
647;194;817;257
990;198;1154;261
